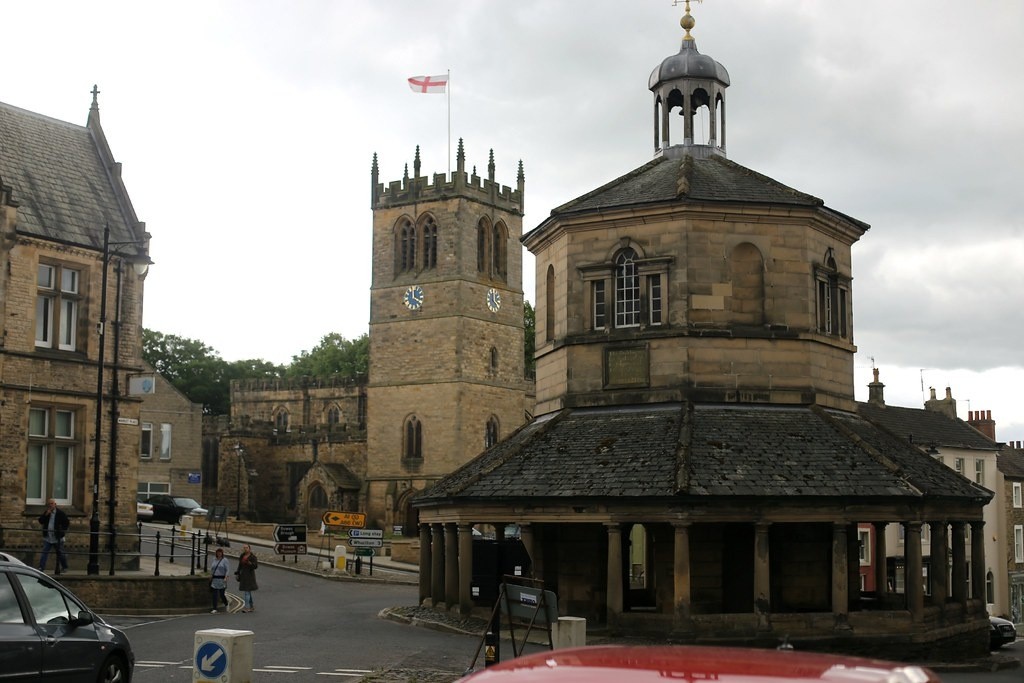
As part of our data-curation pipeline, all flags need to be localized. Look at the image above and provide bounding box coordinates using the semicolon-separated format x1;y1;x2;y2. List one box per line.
408;74;448;93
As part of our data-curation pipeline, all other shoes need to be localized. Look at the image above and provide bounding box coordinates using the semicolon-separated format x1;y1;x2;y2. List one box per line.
242;608;252;613
225;604;230;612
211;610;217;613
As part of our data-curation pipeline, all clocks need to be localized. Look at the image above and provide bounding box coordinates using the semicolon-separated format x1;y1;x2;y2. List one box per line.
486;287;502;313
403;285;425;311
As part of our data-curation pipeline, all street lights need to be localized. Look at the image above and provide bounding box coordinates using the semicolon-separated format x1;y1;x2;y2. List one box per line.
86;221;155;574
234;442;246;519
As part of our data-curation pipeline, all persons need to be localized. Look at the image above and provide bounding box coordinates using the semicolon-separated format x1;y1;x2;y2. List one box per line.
37;499;70;576
234;543;258;612
210;549;229;614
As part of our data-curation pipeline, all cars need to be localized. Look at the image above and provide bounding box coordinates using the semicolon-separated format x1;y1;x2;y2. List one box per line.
144;494;208;526
988;615;1016;648
137;499;154;522
0;561;134;683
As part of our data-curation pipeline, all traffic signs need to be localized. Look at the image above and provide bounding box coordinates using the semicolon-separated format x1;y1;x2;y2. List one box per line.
322;512;365;527
275;545;309;556
273;524;308;544
348;530;383;557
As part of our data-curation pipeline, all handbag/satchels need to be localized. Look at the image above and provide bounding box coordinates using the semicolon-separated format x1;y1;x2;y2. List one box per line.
209;581;212;587
